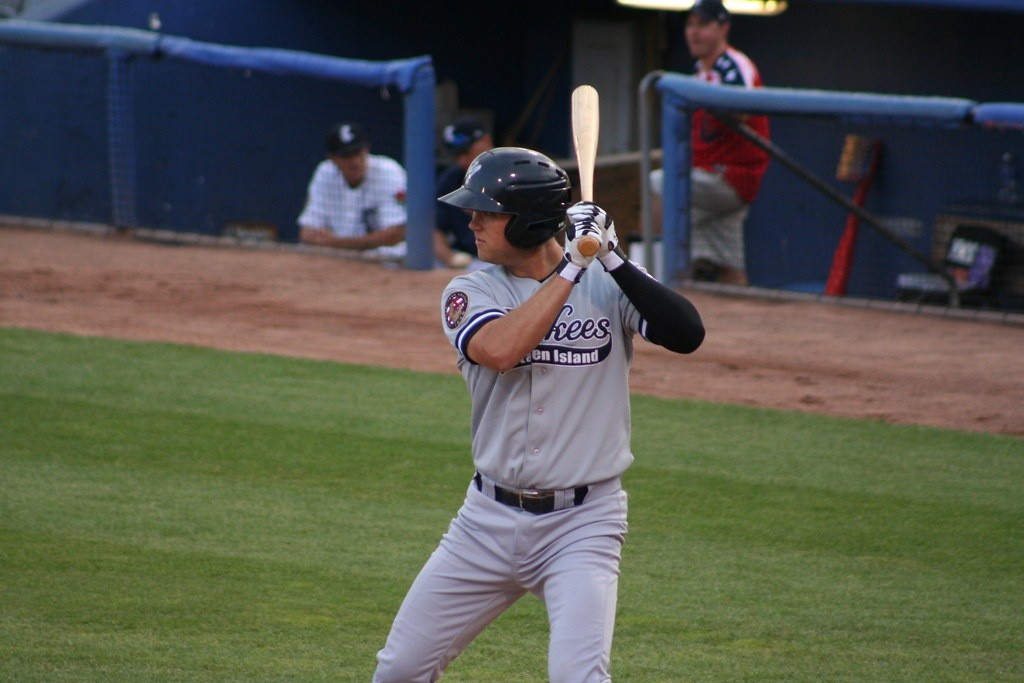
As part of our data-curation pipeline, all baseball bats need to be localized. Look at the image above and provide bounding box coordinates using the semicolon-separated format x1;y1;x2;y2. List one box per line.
823;137;888;296
570;85;601;257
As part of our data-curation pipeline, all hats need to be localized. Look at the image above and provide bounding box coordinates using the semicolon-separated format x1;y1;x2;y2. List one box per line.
442;118;486;155
688;0;729;26
328;122;367;158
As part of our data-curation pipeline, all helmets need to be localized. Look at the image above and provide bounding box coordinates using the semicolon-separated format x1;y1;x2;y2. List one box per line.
437;146;575;250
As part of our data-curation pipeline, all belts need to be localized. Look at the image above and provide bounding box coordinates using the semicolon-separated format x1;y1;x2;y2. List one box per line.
474;472;591;514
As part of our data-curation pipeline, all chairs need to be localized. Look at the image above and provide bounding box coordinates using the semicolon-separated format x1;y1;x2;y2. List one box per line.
897;220;1012;313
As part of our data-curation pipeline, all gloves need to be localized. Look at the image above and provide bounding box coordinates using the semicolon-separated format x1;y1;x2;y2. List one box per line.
554;198;628;284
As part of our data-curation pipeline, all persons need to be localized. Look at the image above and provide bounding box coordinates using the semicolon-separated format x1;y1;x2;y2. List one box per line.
433;116;493;266
298;125;409;255
647;0;771;285
369;146;705;683
